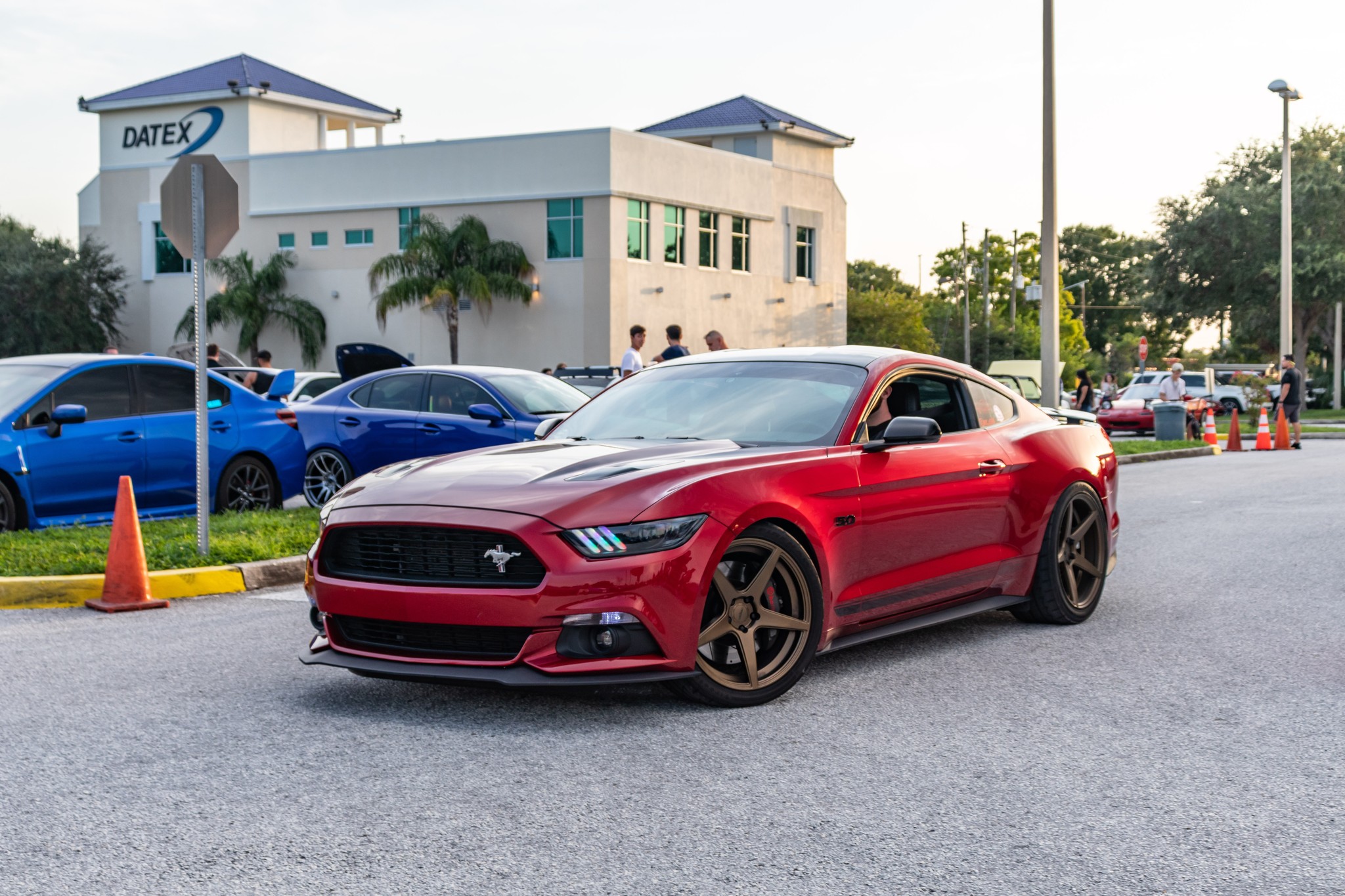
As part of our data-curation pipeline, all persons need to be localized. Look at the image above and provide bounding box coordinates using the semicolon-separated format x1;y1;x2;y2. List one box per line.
542;368;551;376
207;344;229;378
621;324;646;379
558;363;567;368
1075;370;1093;413
1274;354;1302;449
652;325;690;363
103;346;118;354
865;384;894;440
705;330;730;351
242;350;275;395
1101;373;1118;400
1159;363;1198;440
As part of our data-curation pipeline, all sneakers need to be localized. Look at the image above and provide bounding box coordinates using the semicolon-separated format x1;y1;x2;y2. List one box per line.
1291;443;1300;449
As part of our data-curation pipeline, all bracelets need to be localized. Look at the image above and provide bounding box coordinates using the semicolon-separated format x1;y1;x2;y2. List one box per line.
1278;401;1283;403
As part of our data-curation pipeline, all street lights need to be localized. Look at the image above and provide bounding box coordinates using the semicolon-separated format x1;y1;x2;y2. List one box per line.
1266;78;1304;369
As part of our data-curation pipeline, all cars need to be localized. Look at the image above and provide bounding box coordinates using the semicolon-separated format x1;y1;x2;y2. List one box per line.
984;359;1331;426
199;366;623;425
290;364;597;512
298;344;1119;709
0;352;307;536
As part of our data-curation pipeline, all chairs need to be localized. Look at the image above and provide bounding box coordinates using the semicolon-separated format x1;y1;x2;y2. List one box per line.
888;382;923;416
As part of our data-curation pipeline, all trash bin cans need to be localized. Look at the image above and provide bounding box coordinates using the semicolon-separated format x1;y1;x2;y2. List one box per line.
1153;401;1188;440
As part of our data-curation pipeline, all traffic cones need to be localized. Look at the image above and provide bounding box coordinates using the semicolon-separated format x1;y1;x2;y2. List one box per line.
82;474;170;614
